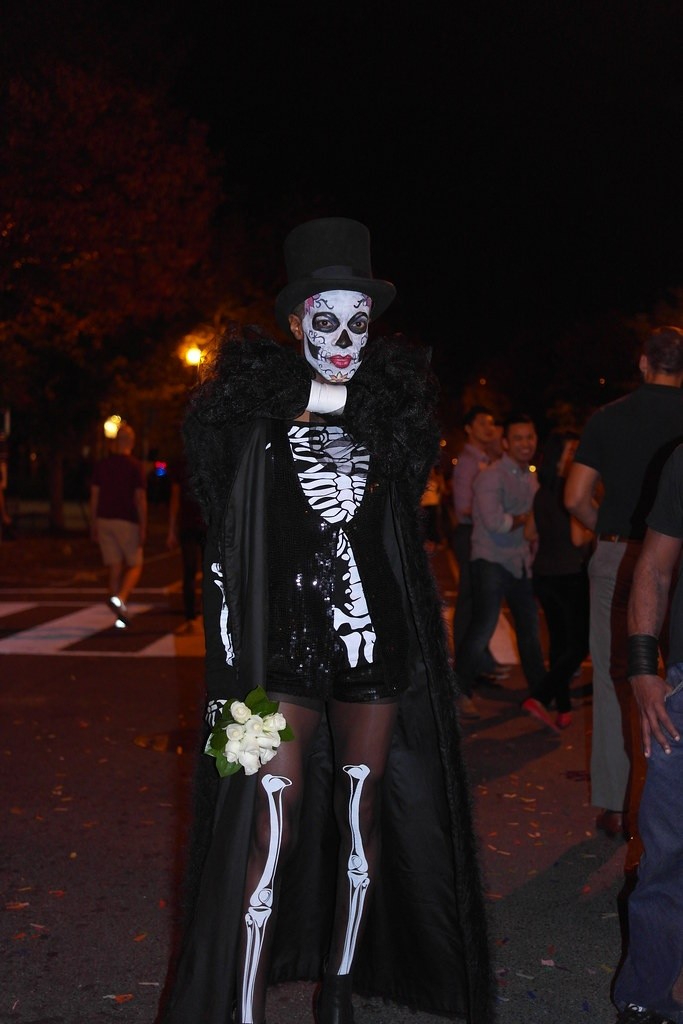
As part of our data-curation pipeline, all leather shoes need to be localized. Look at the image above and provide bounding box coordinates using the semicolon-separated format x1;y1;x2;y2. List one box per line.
318;971;355;1024
596;809;628;838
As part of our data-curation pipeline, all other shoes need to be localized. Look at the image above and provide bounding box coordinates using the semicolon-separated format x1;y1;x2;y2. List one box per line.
107;596;128;630
478;663;512;680
522;698;559;734
184;610;196;620
459;696;480;718
556;710;574;728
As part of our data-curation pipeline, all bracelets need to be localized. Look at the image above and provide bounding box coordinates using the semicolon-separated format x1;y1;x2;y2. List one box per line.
624;634;660;679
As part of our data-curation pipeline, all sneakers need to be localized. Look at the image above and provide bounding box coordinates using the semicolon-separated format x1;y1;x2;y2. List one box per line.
615;1007;669;1024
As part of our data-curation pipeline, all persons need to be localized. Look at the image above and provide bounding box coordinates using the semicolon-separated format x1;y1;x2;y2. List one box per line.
420;404;611;738
164;443;210;620
147;220;508;1024
612;430;683;1024
562;322;682;845
1;426;175;538
89;418;150;630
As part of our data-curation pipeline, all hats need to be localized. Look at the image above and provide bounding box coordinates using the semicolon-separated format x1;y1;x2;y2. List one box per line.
276;217;396;334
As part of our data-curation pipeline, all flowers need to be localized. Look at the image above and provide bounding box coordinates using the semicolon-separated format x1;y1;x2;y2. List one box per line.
204;683;297;778
